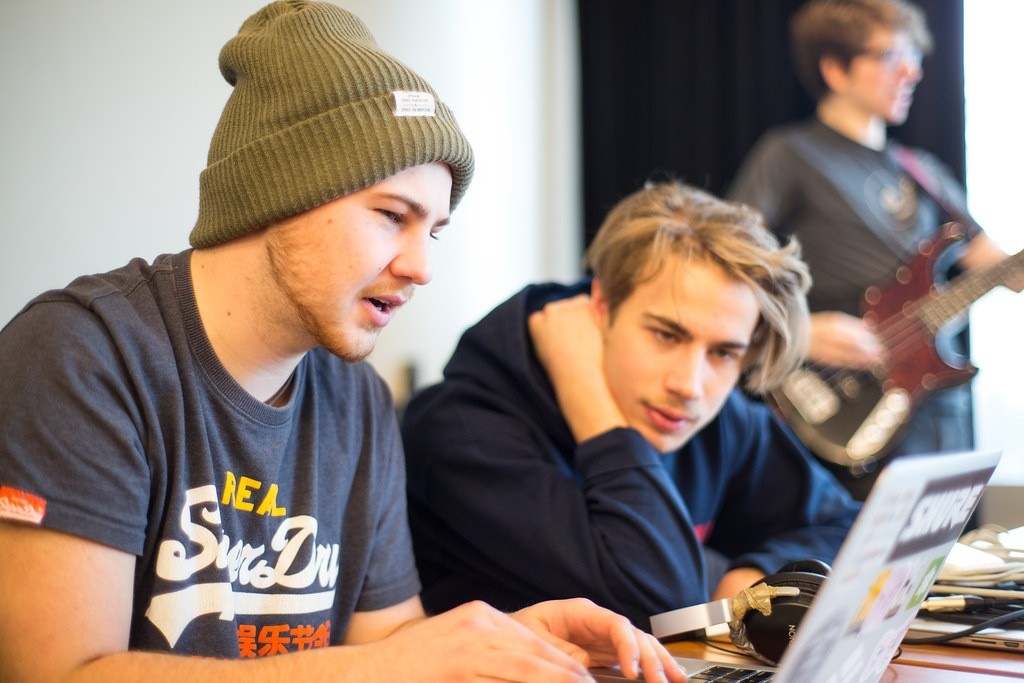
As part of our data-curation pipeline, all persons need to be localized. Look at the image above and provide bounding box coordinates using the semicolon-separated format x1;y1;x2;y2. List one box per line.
401;183;862;638
0;0;689;683
728;0;1024;538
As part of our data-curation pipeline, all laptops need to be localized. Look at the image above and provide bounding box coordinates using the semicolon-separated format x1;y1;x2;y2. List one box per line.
903;614;1024;652
588;450;1001;683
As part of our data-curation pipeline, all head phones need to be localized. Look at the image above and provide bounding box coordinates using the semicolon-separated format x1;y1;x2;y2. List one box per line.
649;557;831;665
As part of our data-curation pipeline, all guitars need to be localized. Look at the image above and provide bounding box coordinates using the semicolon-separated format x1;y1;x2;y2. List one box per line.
755;214;1024;468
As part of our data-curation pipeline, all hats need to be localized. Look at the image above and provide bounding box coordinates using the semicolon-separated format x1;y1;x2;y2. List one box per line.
189;0;475;250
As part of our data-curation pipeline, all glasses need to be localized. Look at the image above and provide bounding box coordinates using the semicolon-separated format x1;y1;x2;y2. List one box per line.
854;47;924;69
